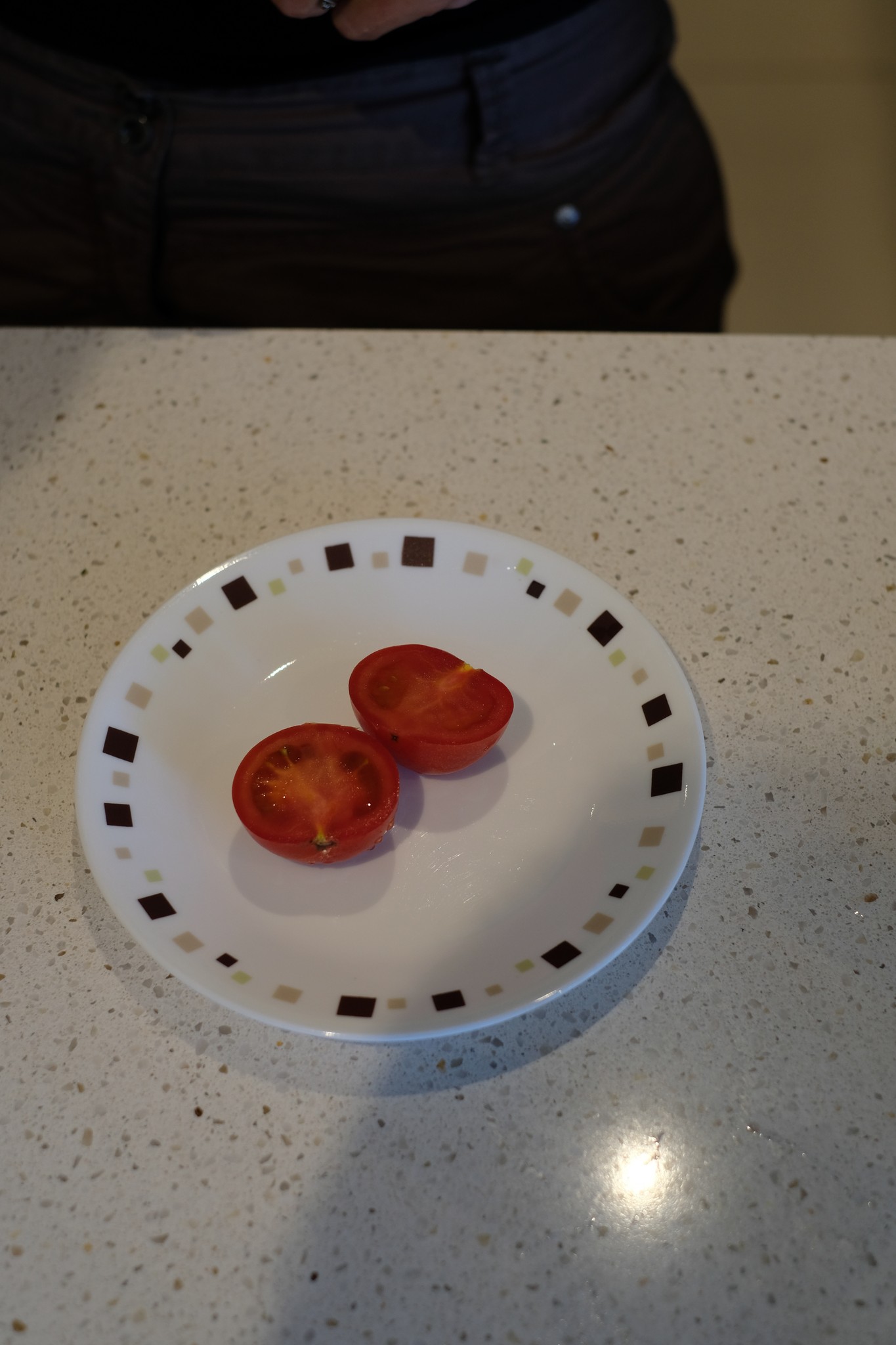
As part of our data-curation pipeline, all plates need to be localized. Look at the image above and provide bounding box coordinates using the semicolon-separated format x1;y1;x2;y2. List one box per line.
69;511;712;1054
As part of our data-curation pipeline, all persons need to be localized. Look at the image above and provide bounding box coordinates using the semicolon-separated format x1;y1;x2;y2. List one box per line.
0;0;737;333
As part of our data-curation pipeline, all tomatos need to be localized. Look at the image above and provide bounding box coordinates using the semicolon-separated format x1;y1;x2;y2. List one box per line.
228;724;399;865
347;645;514;774
